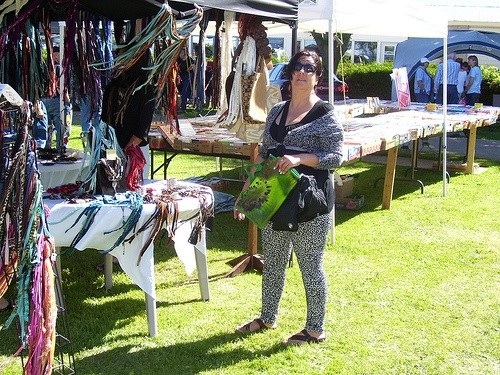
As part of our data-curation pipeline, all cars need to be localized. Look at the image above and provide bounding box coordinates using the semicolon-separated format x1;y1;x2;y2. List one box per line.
269;62;351;103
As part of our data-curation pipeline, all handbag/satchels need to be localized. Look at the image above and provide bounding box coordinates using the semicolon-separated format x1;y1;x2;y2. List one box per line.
271;172;328;230
225;41;284;122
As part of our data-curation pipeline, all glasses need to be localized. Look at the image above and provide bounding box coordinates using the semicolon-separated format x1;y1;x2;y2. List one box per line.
294;62;318;73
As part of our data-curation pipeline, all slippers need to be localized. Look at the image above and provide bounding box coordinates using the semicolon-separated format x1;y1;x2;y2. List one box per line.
234;318;278;334
288;328;327;343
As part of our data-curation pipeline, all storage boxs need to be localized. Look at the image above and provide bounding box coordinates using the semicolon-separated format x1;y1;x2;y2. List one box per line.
150;137;251;155
335;179;365;210
209;181;225;192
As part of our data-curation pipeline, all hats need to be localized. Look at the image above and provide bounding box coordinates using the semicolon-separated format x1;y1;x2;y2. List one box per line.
420;58;430;64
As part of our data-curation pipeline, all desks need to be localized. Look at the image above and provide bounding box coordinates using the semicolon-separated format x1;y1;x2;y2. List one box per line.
41;177;215;337
149;148;251;180
6;146;92;195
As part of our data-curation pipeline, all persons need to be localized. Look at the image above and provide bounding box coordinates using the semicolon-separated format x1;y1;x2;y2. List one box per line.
433;52;460;105
233;50;344;346
175;46;194;114
413;58;433;104
455;57;471;105
460;55;482;107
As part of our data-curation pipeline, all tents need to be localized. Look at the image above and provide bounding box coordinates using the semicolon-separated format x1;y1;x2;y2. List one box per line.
391;30;500;102
173;0;449;196
0;0;335;245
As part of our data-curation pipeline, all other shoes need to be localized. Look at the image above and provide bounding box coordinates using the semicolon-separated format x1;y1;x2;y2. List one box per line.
192;106;203;113
177;108;186;113
